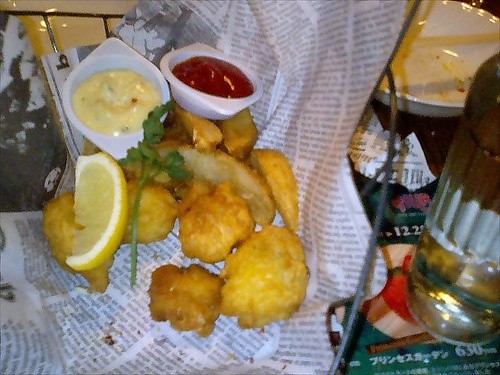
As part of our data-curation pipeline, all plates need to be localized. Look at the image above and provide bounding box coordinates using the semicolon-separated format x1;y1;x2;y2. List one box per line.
371;1;500;118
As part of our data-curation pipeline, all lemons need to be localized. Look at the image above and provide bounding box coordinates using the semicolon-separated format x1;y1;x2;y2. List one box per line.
65;151;128;271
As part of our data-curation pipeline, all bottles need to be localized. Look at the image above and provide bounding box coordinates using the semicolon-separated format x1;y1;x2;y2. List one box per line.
405;53;499;350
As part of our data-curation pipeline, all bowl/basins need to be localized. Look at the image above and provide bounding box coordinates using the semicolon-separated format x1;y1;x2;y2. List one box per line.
63;37;171;159
159;41;264;120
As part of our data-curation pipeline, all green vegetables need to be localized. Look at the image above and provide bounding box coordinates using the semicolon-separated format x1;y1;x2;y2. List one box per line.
117;101;191;285
